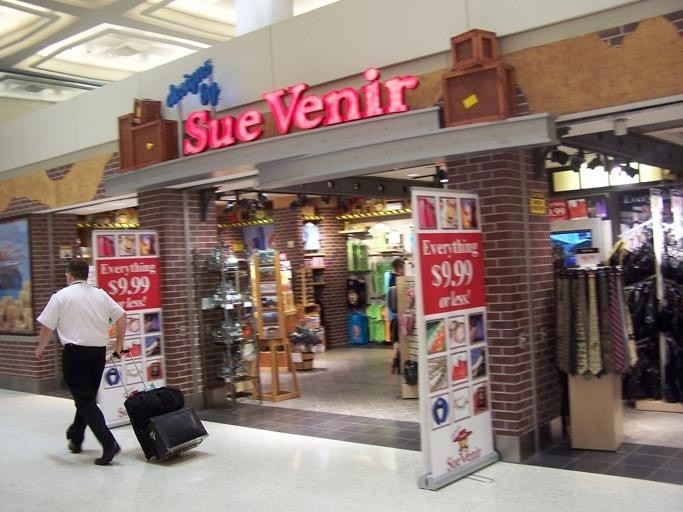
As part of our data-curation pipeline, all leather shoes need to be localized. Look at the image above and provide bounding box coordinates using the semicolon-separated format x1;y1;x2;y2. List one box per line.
66;427;84;453
95;438;121;466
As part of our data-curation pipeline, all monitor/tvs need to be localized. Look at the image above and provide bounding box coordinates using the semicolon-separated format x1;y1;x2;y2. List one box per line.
549;229;592;269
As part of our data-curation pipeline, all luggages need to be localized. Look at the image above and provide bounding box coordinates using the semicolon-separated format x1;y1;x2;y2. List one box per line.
111;349;210;462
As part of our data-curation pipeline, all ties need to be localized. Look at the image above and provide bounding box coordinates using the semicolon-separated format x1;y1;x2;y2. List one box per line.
555;270;638;378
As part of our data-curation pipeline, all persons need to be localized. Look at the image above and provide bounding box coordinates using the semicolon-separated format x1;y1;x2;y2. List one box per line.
34;260;127;465
388;259;405;288
